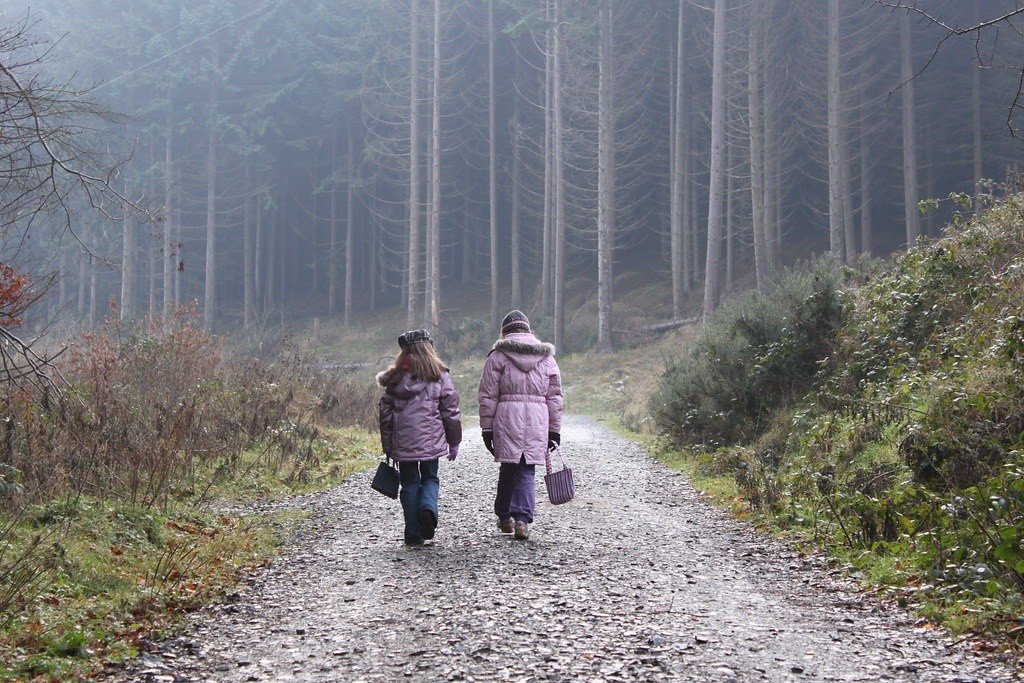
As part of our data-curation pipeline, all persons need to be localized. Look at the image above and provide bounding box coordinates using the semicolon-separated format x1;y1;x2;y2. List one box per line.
374;328;462;546
477;309;565;540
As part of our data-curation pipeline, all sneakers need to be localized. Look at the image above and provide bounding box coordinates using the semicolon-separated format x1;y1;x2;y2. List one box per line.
405;534;424;545
418;511;435;540
497;517;513;533
515;520;529;539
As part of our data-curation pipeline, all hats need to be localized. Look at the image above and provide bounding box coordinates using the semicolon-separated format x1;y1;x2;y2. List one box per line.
501;310;530;334
398;329;433;349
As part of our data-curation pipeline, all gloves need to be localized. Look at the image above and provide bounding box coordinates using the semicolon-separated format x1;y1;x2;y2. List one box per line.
483;437;494;456
447;443;458;461
548;433;560;452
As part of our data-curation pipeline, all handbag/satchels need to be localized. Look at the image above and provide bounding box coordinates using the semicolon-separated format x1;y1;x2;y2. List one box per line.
544;439;576;505
370;457;399;500
